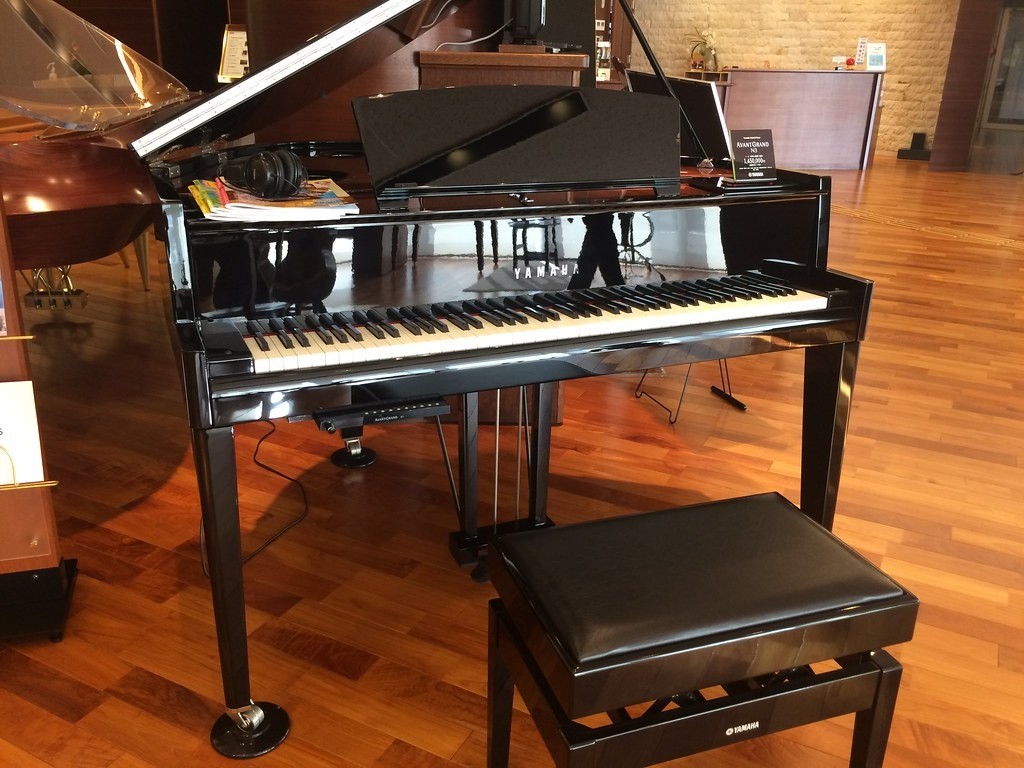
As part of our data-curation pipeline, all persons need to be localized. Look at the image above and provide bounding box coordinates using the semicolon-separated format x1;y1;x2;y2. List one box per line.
566;213;632;289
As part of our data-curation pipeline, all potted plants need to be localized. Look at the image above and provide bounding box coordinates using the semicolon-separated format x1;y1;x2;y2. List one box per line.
686;16;720;72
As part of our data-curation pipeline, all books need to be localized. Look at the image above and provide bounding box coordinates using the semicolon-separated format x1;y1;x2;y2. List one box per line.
687;176;783;195
187;176;360;221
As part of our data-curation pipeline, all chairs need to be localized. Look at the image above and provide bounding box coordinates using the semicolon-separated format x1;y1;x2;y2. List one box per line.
512;217;560;282
195;227;338;313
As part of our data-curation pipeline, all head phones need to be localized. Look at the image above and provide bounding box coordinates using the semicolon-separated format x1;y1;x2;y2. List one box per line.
216;150;304;199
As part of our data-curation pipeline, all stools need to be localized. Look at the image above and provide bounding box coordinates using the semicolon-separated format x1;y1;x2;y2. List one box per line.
486;490;920;768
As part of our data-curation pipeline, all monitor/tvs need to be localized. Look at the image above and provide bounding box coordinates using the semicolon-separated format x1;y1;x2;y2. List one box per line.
511;0;546;45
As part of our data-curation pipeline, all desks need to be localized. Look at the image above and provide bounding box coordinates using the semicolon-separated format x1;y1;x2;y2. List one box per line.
418;52;590;91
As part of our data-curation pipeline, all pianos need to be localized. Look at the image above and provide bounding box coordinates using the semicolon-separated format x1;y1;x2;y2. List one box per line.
124;0;875;760
